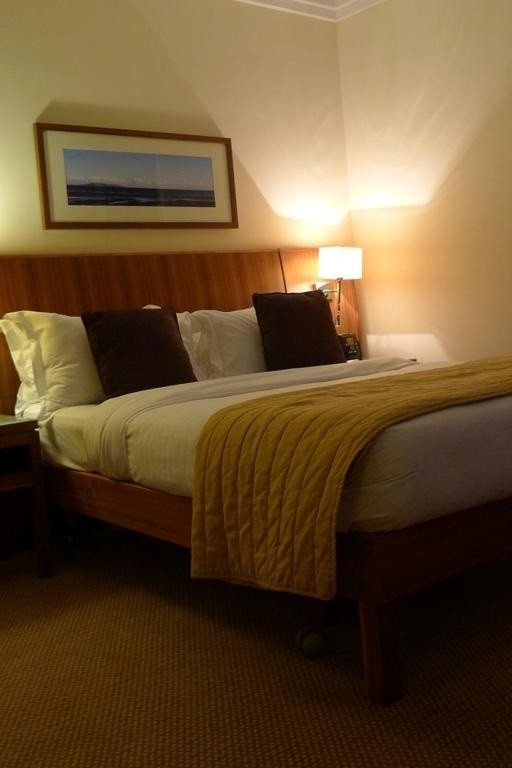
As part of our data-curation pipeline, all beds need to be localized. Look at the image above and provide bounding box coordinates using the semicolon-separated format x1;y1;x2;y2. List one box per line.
1;246;511;702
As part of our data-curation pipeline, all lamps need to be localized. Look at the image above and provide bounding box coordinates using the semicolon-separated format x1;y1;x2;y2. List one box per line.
317;243;362;326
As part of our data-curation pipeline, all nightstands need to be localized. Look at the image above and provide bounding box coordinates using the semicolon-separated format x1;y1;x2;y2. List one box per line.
1;416;51;579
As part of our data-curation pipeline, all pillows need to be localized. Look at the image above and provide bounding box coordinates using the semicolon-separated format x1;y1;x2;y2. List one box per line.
2;309;107;410
189;305;266;382
252;289;347;372
80;306;197;395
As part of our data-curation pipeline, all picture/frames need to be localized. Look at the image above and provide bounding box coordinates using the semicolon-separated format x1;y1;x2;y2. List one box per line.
32;122;239;230
